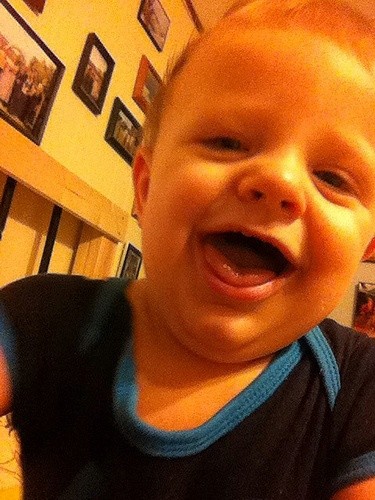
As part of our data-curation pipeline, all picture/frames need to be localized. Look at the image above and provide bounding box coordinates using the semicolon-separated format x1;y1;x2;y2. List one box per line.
105;97;143;165
0;0;66;146
116;242;143;280
137;0;172;53
132;56;162;115
71;33;115;116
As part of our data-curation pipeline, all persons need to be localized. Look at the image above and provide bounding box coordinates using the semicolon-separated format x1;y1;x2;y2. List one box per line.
0;0;375;500
83;68;101;101
114;117;137;155
0;47;44;129
